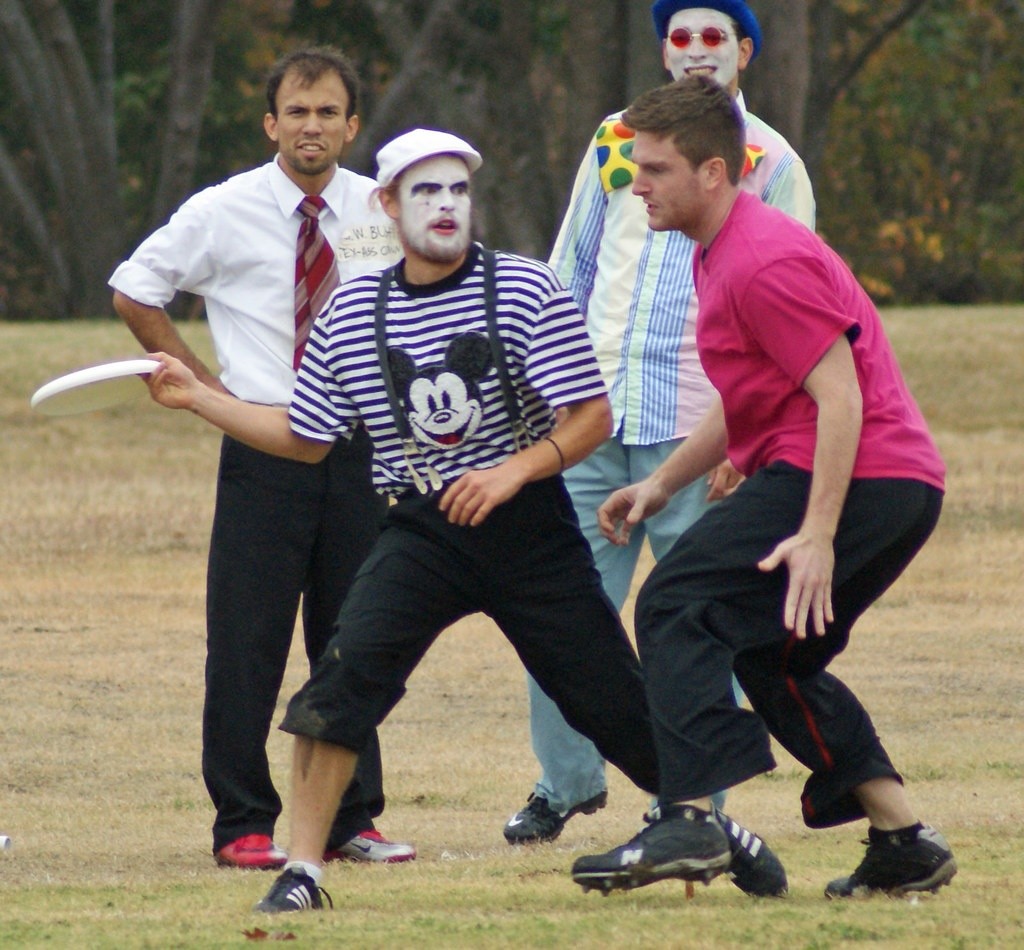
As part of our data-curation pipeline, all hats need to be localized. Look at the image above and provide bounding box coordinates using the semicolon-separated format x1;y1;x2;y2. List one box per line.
375;128;484;187
653;0;763;62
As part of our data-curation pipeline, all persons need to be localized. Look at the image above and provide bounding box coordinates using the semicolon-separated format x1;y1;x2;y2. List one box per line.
569;70;963;900
131;128;792;920
103;46;420;874
499;0;820;847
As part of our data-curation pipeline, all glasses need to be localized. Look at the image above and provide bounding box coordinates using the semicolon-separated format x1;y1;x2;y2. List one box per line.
665;26;739;47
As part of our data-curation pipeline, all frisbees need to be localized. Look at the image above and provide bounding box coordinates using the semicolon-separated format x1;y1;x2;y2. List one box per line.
31;359;165;416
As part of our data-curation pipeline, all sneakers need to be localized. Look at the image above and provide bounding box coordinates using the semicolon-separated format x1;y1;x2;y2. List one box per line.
258;865;321;914
324;828;415;861
717;808;790;897
824;827;955;899
571;803;735;897
503;791;607;843
219;835;289;870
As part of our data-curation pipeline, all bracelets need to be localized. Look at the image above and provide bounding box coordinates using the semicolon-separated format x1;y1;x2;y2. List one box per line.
546;437;565;474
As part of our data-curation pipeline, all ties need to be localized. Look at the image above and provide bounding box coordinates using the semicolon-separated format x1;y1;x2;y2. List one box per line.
291;196;339;372
